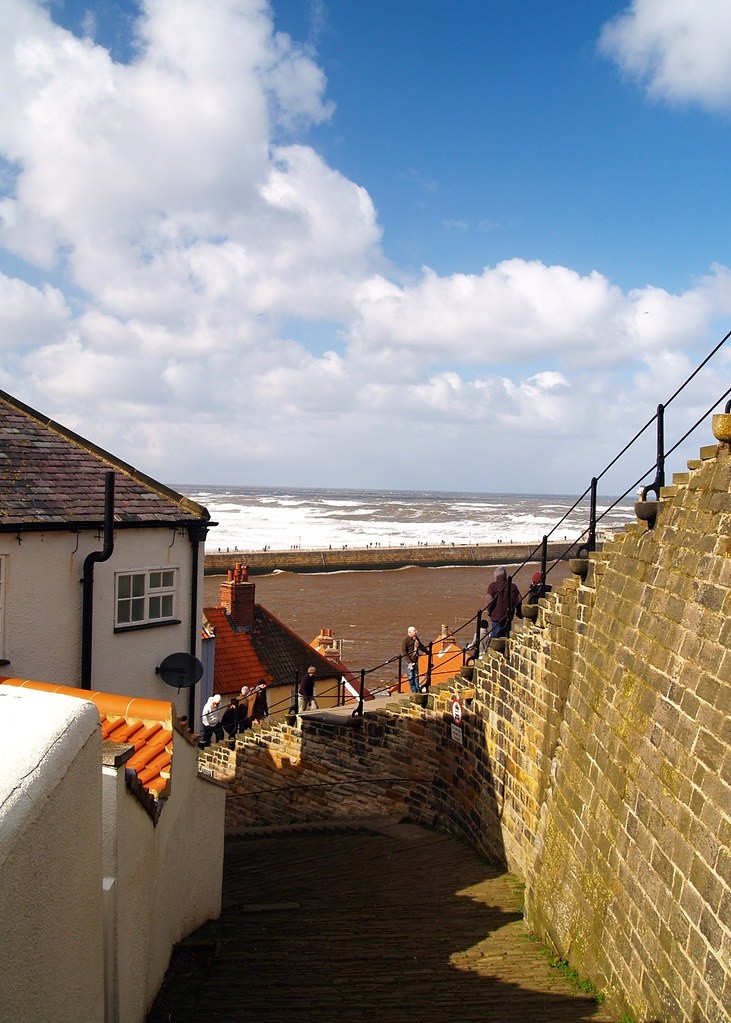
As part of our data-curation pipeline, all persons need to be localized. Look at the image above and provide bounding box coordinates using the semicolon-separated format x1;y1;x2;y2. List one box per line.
527;572;552;605
222;679;269;739
486;567;522;639
400;626;429;693
466;619;491;660
201;694;224;745
297;666;318;713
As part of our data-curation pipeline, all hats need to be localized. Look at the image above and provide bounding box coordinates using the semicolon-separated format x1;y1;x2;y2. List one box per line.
493;566;506;578
213;694;223;703
480;620;488;628
532;572;542;582
231;698;239;706
308;667;317;674
241;686;249;692
258;679;267;685
